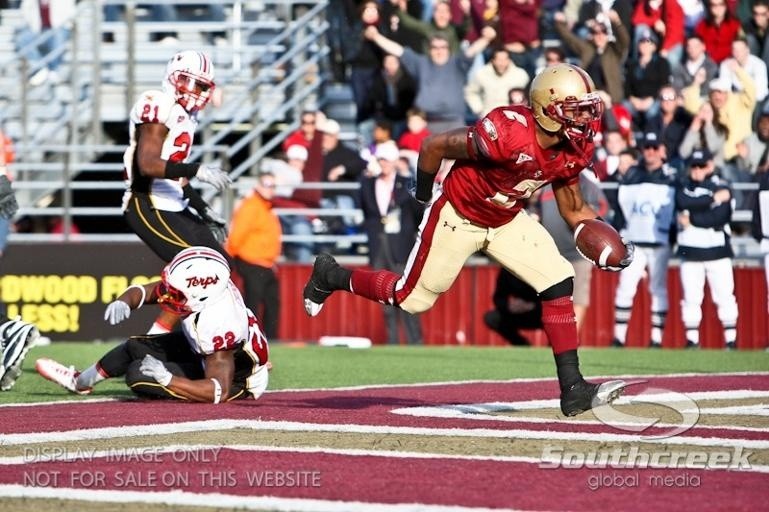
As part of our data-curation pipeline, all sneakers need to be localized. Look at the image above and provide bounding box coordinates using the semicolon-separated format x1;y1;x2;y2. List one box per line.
303;254;339;317
36;358;92;395
0;315;38;391
560;378;625;416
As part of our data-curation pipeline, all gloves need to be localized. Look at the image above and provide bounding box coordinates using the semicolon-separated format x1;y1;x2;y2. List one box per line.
195;164;232;191
104;299;131;326
201;207;229;243
139;353;173;387
599;236;635;273
409;183;442;207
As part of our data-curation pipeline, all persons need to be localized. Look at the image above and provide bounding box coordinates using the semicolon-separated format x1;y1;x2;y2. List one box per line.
0;0;769;416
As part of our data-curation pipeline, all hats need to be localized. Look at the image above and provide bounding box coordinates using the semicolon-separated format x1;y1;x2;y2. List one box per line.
691;148;712;165
641;132;660;146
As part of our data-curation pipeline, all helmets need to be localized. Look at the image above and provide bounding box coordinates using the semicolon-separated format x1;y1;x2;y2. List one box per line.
528;63;604;143
165;49;215;114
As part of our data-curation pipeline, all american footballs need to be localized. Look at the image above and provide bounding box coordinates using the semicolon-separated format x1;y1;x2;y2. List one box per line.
574;218;628;273
